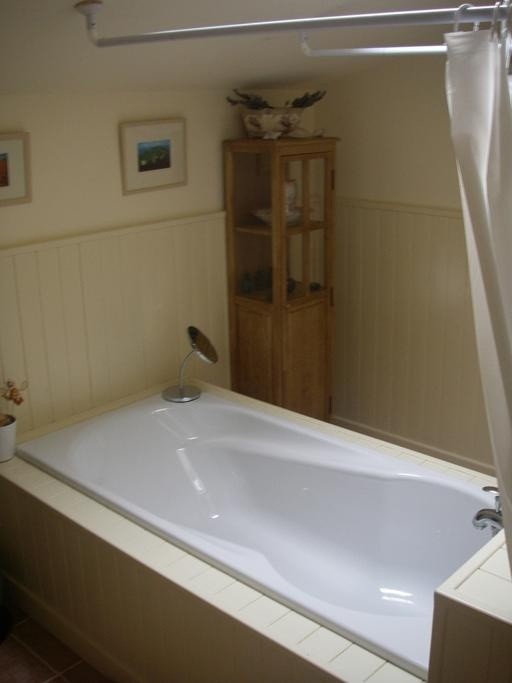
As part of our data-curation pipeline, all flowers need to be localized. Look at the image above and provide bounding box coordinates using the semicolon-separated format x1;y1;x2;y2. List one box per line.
0;378;29;423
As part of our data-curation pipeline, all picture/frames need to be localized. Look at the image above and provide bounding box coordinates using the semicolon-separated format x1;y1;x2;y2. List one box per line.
0;131;32;206
119;116;188;194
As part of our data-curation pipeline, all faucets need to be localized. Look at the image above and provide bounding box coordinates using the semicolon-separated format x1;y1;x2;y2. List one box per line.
471;485;504;538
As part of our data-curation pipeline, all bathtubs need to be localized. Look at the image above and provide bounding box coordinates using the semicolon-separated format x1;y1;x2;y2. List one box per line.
13;392;505;681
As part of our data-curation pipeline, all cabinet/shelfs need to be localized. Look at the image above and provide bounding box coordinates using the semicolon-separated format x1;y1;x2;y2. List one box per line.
222;136;342;423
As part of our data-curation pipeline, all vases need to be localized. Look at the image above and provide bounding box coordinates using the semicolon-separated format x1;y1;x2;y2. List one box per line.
0;414;17;462
